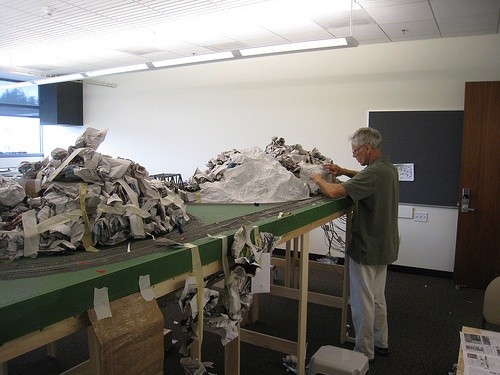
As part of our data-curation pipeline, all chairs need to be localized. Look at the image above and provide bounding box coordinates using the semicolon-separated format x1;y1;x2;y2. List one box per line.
481;276;500;329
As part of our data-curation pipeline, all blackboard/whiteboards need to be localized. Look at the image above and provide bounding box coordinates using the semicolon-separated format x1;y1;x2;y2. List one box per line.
368;110;465;208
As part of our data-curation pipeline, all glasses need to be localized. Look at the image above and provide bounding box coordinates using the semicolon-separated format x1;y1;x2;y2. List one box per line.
352;144;364;154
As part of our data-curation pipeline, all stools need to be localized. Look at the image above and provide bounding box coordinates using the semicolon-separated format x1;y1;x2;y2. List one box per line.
309;345;370;375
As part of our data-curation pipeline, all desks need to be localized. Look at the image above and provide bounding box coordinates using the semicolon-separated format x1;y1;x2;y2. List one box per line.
0;197;354;375
455;325;500;375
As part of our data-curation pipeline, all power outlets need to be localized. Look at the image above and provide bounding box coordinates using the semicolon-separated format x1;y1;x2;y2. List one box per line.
413;213;428;222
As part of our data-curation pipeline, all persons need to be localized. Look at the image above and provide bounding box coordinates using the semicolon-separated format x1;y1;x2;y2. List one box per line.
306;127;400;363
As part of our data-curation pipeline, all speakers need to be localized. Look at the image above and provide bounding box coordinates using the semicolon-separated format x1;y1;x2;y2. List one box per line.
38;80;83;127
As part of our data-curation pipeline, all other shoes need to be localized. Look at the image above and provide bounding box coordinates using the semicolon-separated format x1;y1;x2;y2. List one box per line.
369;359;375;363
375;346;390;356
